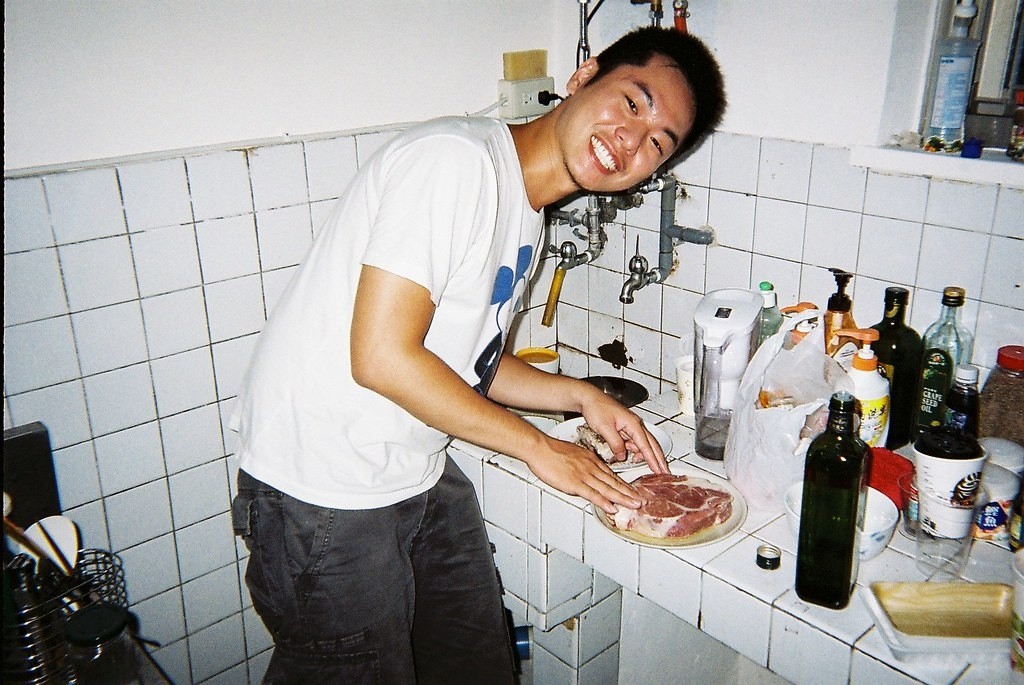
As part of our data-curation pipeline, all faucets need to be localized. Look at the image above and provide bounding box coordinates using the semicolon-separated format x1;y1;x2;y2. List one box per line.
540;240;578;327
618;234;660;305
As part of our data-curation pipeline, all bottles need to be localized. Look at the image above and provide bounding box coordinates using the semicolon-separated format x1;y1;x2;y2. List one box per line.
65;604;142;685
1009;476;1024;552
756;281;783;351
923;0;983;152
794;391;870;610
939;364;980;442
911;287;974;443
867;286;921;452
978;345;1024;446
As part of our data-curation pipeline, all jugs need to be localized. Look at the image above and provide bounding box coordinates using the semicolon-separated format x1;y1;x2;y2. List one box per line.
693;288;764;463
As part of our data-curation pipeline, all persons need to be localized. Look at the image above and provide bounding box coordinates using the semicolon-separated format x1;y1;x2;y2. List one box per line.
227;27;727;685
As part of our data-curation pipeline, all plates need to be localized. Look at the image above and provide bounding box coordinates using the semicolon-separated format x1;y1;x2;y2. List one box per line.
547;417;672;472
594;471;748;549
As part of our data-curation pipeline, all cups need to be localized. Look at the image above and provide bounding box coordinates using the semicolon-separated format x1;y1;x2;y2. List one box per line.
912;474;989;581
1010;548;1024;676
911;442;988;538
516;347;560;375
896;471;919;542
675;356;693;415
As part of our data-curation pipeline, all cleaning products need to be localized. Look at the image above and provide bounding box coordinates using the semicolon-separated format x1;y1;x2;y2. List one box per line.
833;330;891;448
782;300;819;344
759;282;784;342
822;263;861;353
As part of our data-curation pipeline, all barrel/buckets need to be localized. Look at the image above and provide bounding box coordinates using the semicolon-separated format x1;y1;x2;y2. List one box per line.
563;376;649;421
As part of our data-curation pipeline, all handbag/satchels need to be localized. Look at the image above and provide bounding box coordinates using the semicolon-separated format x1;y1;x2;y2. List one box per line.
723;310;855;514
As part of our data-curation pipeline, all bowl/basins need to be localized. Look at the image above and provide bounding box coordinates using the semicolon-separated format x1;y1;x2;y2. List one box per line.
785;480;899;563
858;582;1015;662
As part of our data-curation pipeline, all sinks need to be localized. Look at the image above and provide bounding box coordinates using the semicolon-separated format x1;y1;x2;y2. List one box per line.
447;371;649;485
